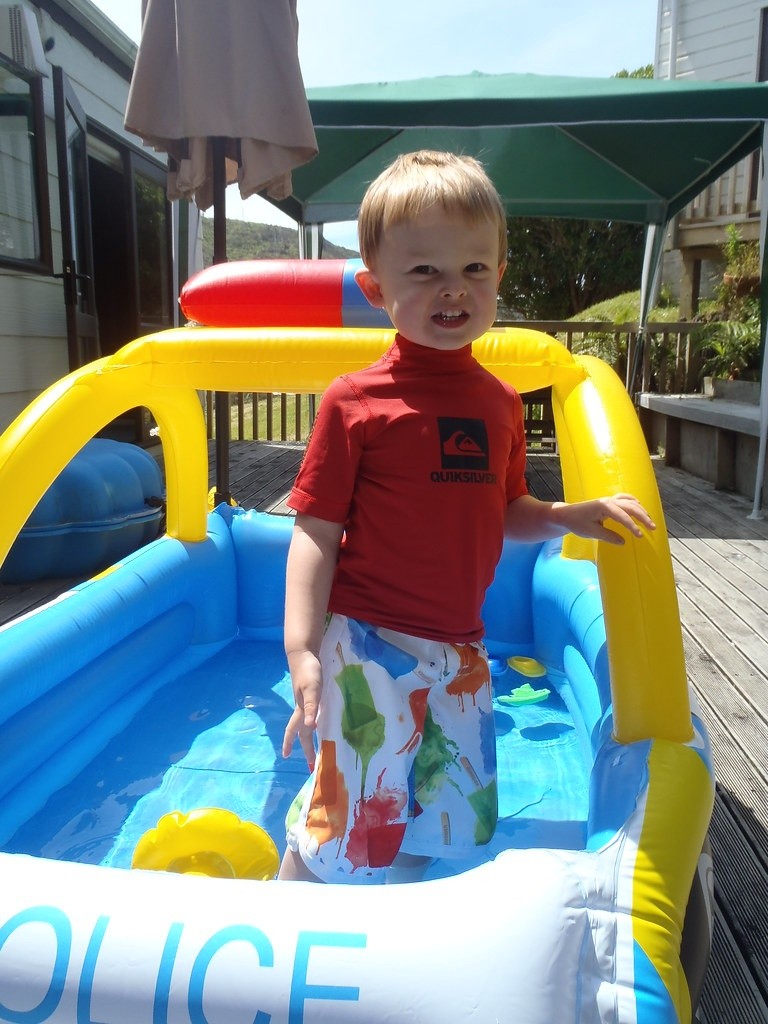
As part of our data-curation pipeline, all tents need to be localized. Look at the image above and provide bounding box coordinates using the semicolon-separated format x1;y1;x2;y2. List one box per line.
254;68;768;523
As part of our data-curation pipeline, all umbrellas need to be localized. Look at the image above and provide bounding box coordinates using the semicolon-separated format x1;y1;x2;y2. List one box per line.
125;0;318;504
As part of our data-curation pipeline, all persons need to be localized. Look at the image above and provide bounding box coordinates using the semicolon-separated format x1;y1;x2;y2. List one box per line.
276;149;656;885
